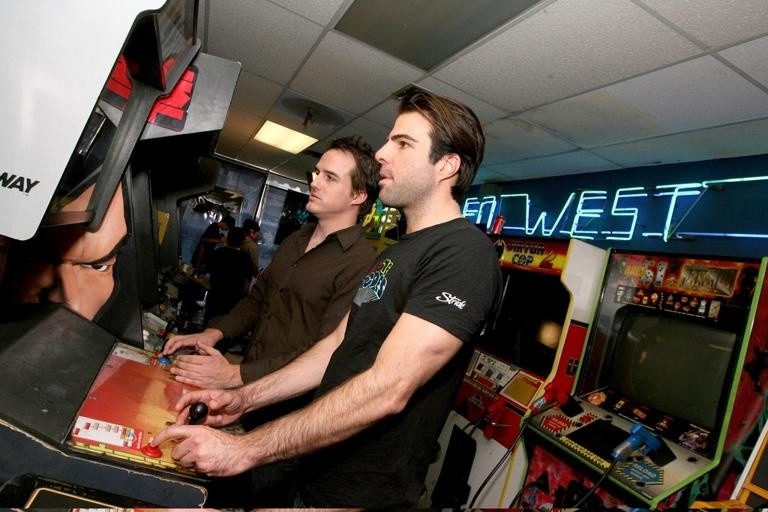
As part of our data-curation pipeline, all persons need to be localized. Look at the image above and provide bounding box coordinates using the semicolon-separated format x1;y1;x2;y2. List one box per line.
0;161;132;322
162;133;381;433
149;86;504;512
190;216;261;330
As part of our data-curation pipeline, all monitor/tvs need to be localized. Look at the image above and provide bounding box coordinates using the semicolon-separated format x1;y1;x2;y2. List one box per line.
597;303;736;434
481;272;569;378
46;55;133;216
